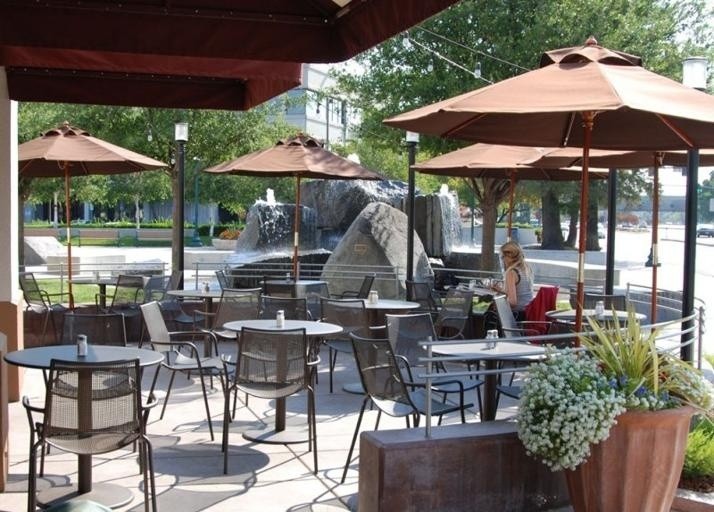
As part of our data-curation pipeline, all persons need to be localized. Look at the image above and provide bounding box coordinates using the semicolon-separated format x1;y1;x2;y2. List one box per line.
485;241;536;336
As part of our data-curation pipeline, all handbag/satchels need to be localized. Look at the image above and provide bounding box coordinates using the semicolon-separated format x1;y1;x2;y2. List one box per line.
434;270;459;291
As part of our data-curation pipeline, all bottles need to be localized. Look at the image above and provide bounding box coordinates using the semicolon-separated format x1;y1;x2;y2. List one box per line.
485;330;493;349
205;283;209;291
370;291;374;303
286;273;290;283
202;282;206;293
374;291;378;303
97;272;100;282
277;310;284;327
493;330;498;348
93;271;96;281
77;334;87;356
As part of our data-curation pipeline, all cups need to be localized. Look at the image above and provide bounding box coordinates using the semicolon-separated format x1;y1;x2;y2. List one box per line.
470;280;476;291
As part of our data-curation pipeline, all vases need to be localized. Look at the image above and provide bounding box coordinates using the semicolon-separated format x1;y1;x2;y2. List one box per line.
563;362;702;511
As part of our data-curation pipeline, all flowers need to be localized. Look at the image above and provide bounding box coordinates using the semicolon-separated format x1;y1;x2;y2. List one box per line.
509;300;714;473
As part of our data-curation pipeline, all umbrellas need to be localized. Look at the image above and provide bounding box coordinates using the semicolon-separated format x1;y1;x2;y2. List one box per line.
410;142;609;241
518;141;713;347
16;120;168;312
203;131;382;280
383;37;712;359
